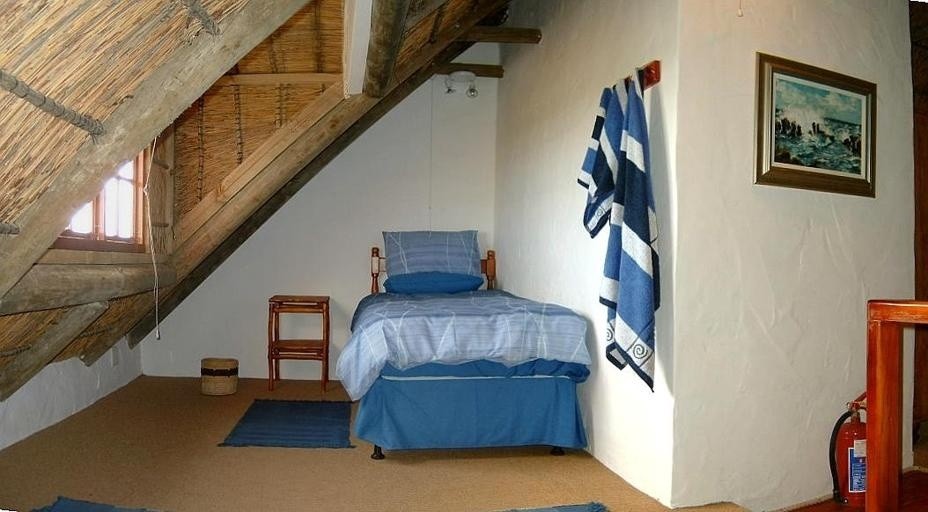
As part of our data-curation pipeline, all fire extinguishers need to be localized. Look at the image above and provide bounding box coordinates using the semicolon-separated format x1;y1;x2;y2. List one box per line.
829;392;868;512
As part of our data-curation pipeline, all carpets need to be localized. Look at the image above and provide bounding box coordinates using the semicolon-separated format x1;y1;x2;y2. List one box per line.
217;398;357;448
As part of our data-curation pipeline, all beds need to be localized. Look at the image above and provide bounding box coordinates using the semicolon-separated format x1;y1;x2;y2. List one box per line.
356;246;588;457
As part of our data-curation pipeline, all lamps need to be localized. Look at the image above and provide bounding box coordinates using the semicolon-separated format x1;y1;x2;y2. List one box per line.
444;71;480;100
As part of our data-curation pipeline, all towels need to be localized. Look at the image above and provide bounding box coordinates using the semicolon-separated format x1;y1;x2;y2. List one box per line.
576;66;662;394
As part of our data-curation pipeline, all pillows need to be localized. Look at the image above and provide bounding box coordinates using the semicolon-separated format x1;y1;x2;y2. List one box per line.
382;229;480;294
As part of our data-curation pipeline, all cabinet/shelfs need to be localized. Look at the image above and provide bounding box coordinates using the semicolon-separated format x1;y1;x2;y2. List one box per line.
267;294;330;392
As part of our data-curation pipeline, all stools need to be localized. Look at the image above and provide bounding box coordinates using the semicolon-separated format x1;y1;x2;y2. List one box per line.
200;357;239;396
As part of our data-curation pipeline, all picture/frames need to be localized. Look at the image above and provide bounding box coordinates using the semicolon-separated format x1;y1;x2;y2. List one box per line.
753;51;878;199
